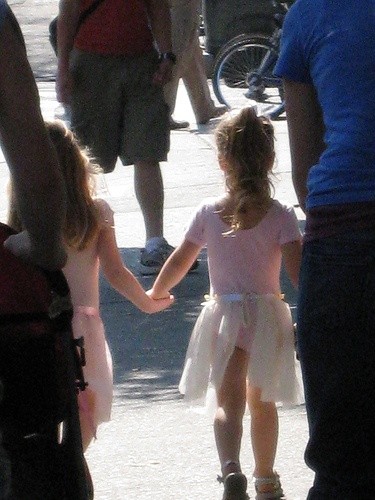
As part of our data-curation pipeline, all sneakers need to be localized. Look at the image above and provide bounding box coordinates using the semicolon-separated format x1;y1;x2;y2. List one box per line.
138;244;199;275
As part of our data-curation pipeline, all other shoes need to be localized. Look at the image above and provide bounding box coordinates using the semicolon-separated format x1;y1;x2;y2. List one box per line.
168;120;189;130
196;105;228;124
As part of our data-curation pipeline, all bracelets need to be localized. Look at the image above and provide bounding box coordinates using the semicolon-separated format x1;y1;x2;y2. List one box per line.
161;50;177;66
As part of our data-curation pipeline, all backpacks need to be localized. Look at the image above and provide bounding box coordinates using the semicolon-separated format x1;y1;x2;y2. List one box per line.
48;17;57;56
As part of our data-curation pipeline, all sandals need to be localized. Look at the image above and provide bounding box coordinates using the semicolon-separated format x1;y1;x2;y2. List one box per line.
252;470;285;500
217;461;247;500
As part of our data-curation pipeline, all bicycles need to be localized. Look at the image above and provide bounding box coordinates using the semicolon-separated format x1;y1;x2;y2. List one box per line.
212;13;289;120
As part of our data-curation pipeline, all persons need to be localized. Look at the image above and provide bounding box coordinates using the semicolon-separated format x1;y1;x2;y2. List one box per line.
153;0;230;130
0;0;69;273
6;118;176;453
57;1;205;276
143;104;306;500
271;1;375;500
